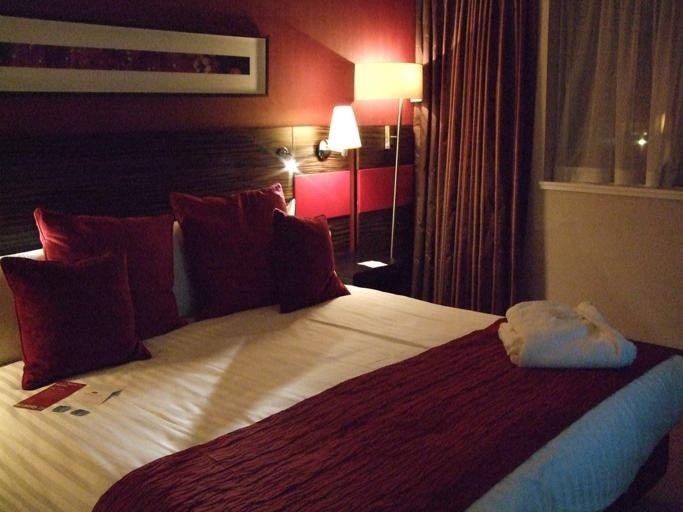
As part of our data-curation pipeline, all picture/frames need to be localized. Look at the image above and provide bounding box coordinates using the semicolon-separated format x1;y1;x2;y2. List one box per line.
0;16;269;98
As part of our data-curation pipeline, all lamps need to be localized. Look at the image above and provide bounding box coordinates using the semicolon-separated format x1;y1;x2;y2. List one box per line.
353;62;423;260
317;105;363;160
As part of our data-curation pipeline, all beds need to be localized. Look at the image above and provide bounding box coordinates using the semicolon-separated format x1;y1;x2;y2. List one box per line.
1;125;683;510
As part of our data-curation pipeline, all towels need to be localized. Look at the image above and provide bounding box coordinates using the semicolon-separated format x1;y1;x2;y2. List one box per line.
498;303;636;370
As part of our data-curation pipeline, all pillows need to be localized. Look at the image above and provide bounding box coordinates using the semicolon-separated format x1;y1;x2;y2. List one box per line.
168;185;292;322
33;206;189;340
269;204;349;315
0;245;154;389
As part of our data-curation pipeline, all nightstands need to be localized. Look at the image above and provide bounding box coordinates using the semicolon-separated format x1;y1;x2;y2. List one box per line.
335;261;411;296
